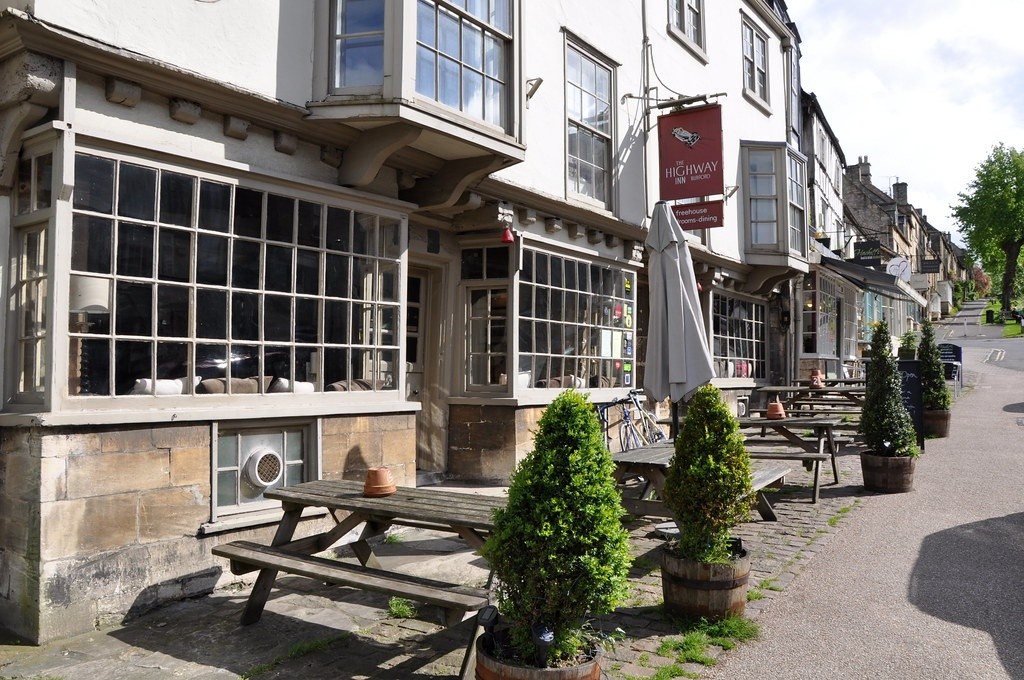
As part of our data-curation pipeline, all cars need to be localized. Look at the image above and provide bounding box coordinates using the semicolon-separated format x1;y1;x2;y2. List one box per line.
123;344;305;396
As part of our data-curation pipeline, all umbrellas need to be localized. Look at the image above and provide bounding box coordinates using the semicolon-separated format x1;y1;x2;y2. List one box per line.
643;200;716;449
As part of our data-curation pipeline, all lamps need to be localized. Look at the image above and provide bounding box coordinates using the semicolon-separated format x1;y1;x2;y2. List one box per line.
499;224;515;243
69;275;110;396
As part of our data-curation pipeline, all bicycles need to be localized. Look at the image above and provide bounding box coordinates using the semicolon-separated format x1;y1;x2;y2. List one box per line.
594;386;669;453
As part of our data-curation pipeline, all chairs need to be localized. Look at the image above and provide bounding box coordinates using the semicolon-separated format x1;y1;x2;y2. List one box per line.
940;362;961;398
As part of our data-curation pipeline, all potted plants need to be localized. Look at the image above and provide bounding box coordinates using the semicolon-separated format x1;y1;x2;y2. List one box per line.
857;317;920;494
916;317;951;437
476;388;628;679
897;332;919;359
658;383;751;621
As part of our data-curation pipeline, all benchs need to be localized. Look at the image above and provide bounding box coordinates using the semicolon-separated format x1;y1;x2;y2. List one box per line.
655;392;866;539
211;514;496;680
998;311;1019;323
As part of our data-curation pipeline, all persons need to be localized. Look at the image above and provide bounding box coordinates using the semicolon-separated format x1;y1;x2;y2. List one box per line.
1001;307;1006;320
1011;308;1024;326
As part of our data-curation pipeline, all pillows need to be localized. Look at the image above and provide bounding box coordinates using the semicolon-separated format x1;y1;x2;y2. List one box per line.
197;375;274;394
127;376;201;396
267;378;317;394
325;379;386;392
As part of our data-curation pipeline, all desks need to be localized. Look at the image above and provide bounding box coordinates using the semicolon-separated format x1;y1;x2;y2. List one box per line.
608;377;866;500
941;361;963;390
241;478;508;680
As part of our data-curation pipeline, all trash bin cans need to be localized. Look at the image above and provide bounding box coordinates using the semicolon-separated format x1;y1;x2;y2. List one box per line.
986;309;994;323
